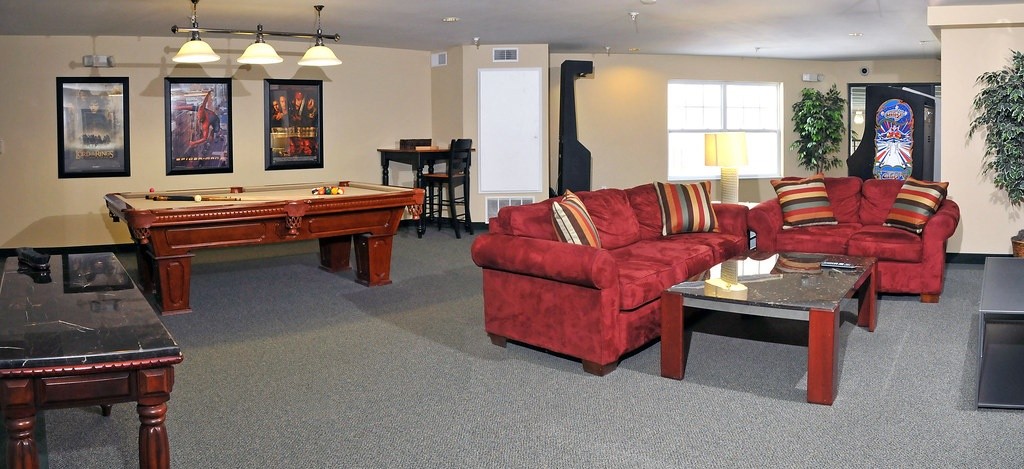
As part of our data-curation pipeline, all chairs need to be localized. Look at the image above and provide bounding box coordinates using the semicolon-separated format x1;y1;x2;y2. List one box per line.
420;139;473;239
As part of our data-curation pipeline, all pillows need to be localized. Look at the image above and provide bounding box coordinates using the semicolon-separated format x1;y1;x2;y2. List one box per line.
653;181;721;236
882;177;949;234
550;188;602;249
770;173;838;230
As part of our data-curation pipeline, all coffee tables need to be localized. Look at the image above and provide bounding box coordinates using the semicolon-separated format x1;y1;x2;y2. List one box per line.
661;251;878;406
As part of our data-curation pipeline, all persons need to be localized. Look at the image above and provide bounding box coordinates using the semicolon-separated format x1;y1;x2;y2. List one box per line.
173;105;220;147
77;90;111;114
272;90;317;120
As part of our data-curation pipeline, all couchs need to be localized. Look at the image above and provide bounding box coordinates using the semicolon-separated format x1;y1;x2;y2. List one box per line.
472;183;750;376
747;177;960;304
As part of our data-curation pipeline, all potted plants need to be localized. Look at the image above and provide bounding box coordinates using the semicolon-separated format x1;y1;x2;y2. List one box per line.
968;48;1024;258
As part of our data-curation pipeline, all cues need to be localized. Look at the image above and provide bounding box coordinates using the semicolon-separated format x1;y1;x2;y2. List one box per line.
145;195;279;201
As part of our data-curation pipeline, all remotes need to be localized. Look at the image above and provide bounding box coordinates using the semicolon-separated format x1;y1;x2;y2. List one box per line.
820;262;856;269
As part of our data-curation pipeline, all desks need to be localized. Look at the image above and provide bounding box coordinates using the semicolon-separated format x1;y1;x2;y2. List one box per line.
377;148;476;239
0;251;183;469
103;180;426;316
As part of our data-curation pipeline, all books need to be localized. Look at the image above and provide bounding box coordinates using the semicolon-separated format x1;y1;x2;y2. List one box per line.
416;146;440;150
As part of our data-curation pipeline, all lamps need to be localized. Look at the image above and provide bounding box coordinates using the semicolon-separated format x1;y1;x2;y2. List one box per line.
171;0;342;66
83;55;116;68
704;132;749;205
803;73;825;83
704;258;748;300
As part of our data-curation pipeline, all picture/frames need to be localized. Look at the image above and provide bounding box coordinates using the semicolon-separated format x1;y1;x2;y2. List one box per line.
264;79;324;171
163;77;233;175
55;77;131;179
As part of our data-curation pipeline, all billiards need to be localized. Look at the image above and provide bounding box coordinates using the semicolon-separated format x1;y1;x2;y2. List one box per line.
313;185;345;194
150;188;154;193
195;195;201;202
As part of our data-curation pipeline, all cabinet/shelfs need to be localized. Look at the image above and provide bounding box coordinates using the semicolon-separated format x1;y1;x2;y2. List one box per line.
976;257;1024;409
